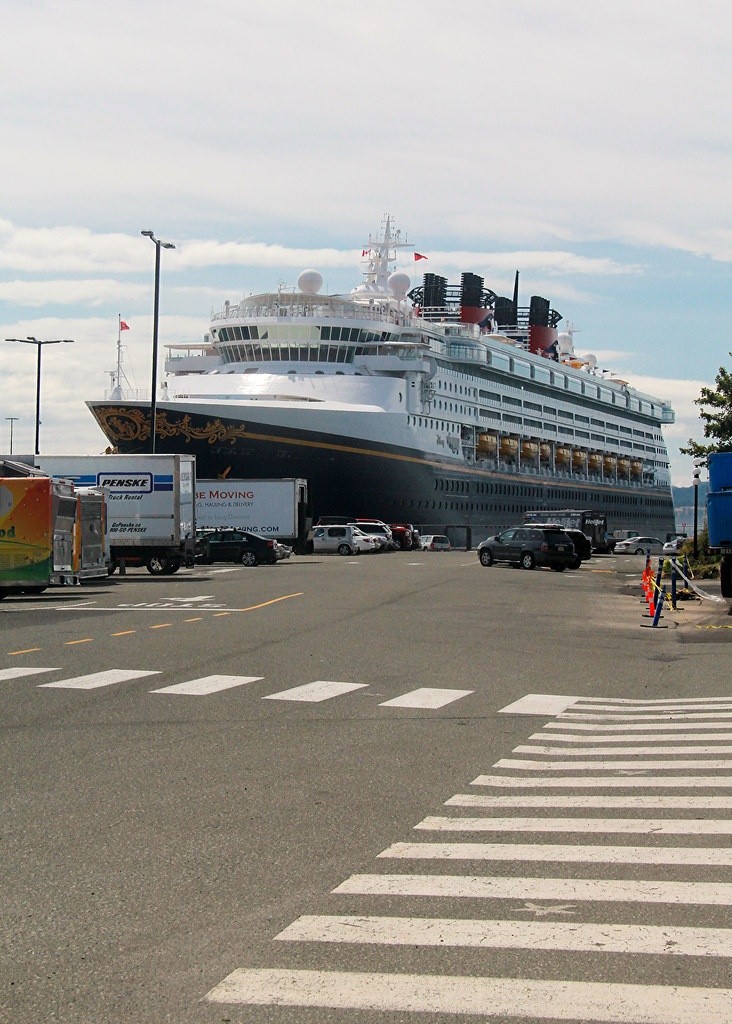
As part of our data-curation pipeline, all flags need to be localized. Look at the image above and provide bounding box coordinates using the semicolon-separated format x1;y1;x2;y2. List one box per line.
414;252;429;261
120;321;130;331
